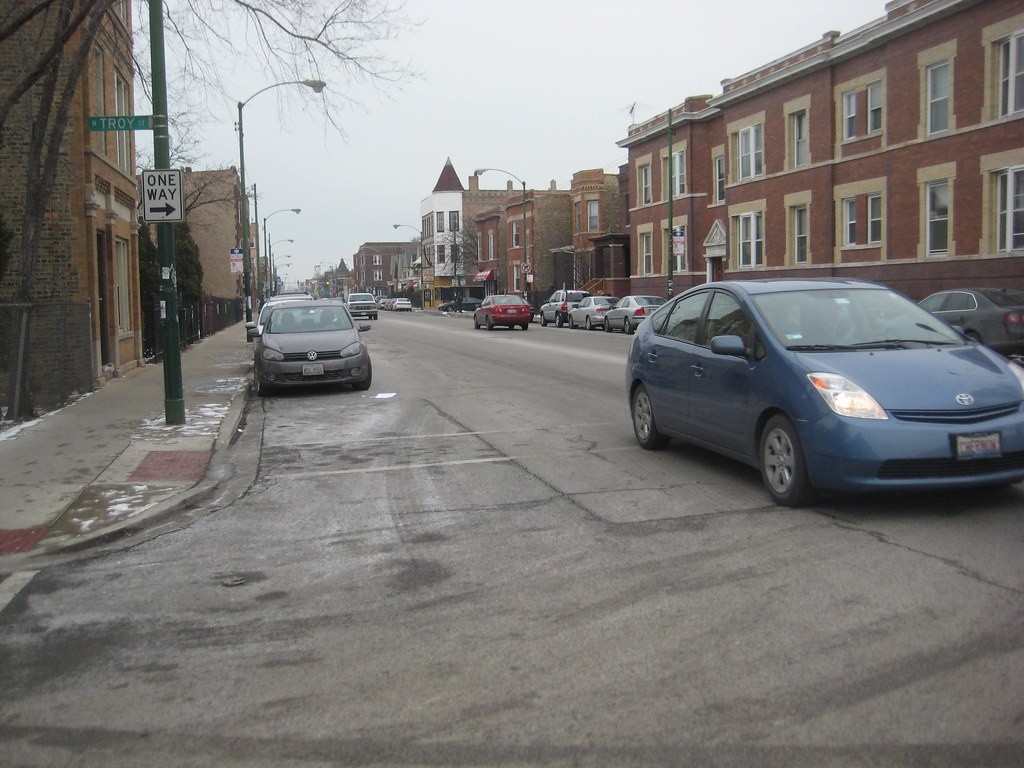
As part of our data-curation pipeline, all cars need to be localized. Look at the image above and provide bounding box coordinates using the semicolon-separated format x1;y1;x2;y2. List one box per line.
522;299;534;322
473;295;531;331
603;296;668;334
625;276;1024;507
918;287;1024;356
344;293;378;320
247;301;372;397
377;298;412;311
246;300;337;350
540;290;592;328
568;297;620;330
442;297;482;312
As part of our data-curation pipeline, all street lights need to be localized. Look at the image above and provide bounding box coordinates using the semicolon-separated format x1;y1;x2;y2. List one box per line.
475;169;528;301
269;233;294;296
238;81;327;343
264;209;301;298
393;224;423;310
272;253;291;296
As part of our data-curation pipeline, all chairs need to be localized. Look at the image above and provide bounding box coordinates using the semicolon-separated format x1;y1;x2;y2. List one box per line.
301;318;315;328
281;313;295;330
318;310;339;330
